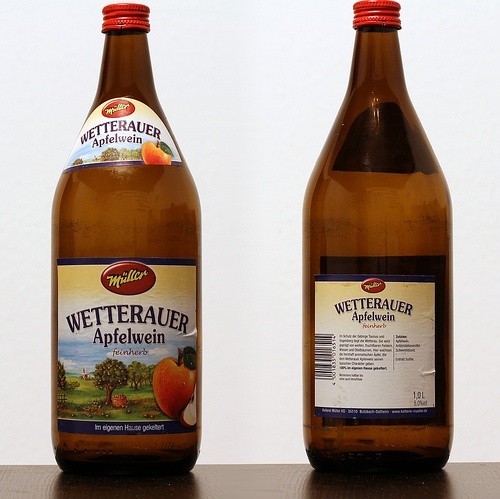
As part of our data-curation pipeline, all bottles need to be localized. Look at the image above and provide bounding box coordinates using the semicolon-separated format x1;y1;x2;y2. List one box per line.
303;0;454;475
53;0;202;476
